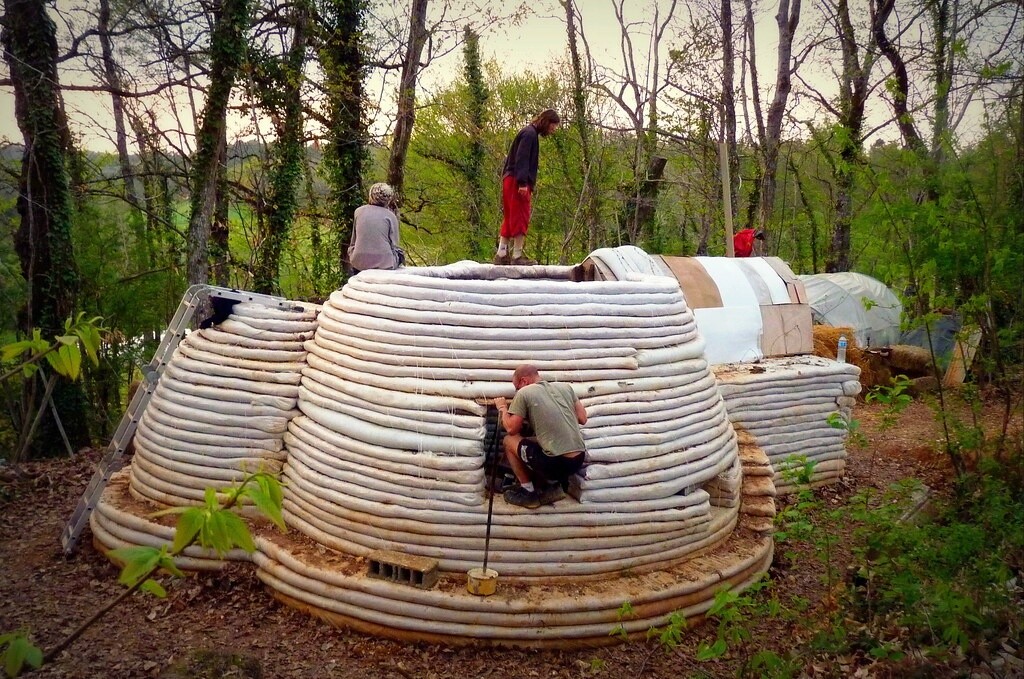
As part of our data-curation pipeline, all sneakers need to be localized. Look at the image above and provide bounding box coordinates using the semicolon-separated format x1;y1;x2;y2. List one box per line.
538;482;566;505
504;487;541;509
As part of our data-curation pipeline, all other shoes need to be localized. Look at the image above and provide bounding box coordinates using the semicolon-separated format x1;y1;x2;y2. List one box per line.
493;255;539;265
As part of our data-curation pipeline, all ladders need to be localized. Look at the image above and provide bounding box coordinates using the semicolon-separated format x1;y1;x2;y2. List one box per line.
59;282;308;558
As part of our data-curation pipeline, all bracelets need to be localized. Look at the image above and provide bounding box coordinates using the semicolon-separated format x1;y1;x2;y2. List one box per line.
497;404;508;410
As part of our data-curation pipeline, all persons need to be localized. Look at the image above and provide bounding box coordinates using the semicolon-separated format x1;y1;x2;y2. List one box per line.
490;109;560;265
347;182;405;275
494;363;588;511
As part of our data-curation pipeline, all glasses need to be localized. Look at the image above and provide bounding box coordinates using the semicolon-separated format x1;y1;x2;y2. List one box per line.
515;377;524;392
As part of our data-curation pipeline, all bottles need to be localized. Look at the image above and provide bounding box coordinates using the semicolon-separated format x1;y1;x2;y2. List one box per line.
836;334;846;362
